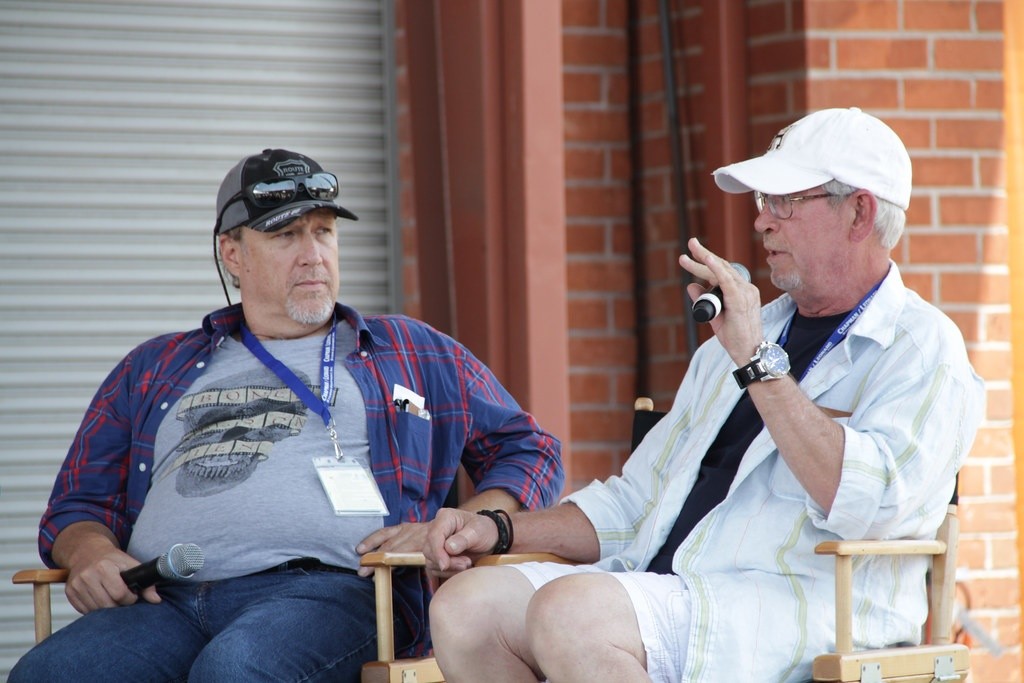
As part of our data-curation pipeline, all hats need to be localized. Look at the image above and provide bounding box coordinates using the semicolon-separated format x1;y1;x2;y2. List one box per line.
710;105;914;211
215;148;362;234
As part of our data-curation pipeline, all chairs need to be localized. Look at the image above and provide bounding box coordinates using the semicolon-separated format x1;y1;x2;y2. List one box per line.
475;504;971;683
12;551;445;683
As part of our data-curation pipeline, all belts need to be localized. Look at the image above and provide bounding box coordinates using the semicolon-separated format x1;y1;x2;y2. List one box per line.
266;556;322;576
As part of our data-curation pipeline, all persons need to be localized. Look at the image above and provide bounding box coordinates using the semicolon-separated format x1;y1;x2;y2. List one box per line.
422;105;990;683
8;148;565;683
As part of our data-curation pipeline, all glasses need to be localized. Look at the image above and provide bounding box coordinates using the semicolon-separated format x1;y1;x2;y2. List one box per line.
753;190;854;220
213;170;339;233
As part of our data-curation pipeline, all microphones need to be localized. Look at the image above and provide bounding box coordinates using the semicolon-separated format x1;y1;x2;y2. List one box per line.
119;543;205;594
692;262;751;323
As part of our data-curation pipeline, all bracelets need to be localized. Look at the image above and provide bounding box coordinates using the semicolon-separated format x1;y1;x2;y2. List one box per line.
476;508;515;555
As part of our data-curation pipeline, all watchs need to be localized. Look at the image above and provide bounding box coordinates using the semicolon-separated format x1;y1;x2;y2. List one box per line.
732;340;792;390
